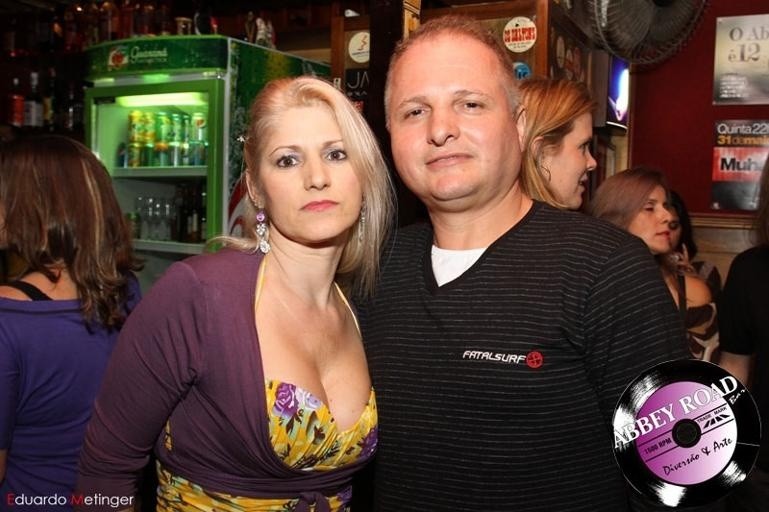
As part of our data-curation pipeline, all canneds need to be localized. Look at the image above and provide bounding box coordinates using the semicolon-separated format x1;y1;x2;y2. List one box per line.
118;111;209;167
124;212;142;239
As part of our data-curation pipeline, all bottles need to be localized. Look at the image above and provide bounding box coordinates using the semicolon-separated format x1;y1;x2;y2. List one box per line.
8;66;83;140
1;0;176;59
135;177;206;244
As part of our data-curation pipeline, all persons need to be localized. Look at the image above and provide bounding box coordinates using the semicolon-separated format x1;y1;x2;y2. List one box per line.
331;15;699;508
714;151;768;404
581;164;721;366
0;127;143;510
77;75;395;510
665;188;722;295
515;74;599;212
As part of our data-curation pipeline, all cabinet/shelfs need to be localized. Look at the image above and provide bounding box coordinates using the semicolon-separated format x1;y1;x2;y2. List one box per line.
414;0;635;222
0;1;385;141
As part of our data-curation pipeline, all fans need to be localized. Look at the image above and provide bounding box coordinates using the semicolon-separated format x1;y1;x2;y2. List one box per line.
586;0;708;66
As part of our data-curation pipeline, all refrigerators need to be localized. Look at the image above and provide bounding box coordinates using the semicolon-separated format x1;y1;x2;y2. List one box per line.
73;29;336;302
